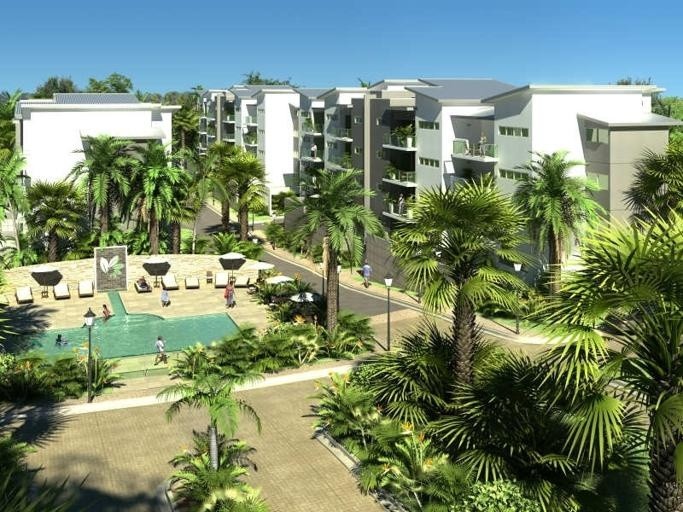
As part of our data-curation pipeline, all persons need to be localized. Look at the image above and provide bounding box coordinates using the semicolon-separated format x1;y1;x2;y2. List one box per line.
396;193;405;216
361;260;373;288
152;335;166;366
159;287;169;307
224;281;236;308
54;333;63;346
309;143;317;160
476;130;488;158
101;304;110;319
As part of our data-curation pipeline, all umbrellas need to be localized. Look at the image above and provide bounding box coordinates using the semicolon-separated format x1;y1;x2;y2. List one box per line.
219;253;245;281
142;256;170;289
29;264;62;299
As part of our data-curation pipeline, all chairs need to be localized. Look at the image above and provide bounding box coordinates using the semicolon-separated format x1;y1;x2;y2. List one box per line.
10;265;253;305
